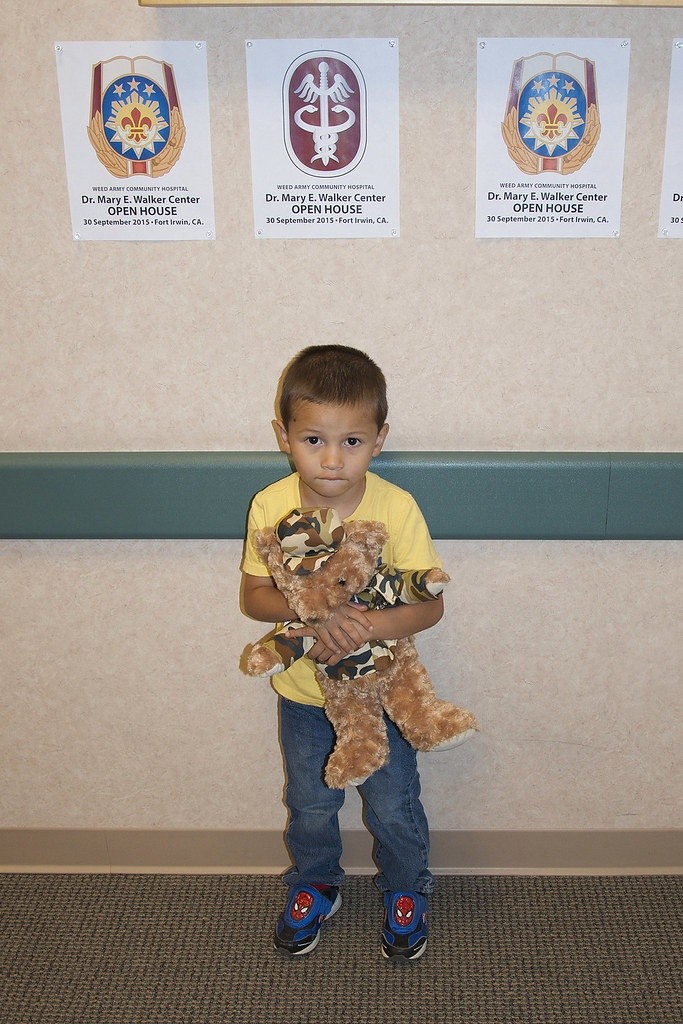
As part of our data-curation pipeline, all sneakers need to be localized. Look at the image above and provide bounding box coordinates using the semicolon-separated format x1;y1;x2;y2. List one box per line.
382;890;428;961
274;884;343;954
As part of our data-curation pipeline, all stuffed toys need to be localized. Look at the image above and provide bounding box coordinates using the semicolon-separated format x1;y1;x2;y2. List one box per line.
247;506;480;789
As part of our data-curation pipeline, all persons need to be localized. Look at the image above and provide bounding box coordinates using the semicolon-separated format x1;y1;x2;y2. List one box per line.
242;344;444;960
